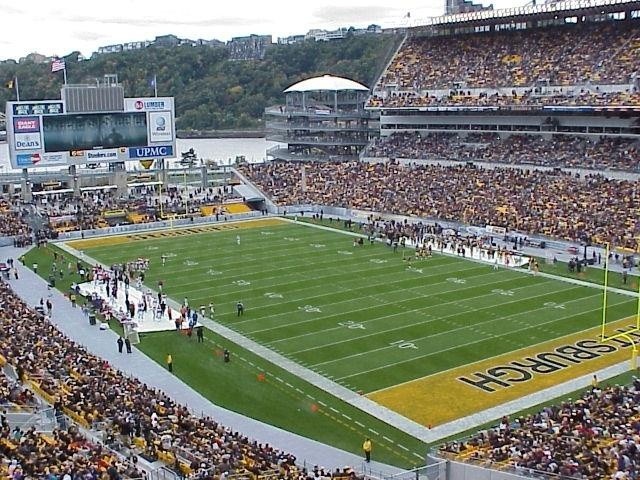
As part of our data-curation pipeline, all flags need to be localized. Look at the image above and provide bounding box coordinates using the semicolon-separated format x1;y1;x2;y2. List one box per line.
149;79;156;88
5;77;16;89
51;59;66;72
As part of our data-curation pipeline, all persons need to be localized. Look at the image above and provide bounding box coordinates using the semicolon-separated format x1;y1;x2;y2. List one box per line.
363;439;372;464
433;371;640;479
363;16;640;283
1;157;364;479
